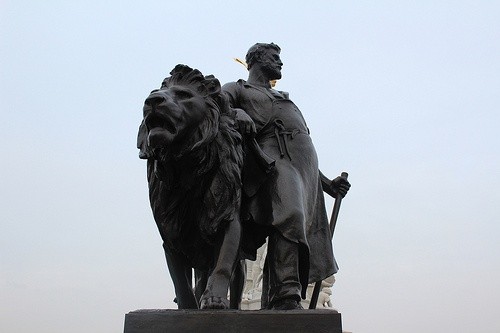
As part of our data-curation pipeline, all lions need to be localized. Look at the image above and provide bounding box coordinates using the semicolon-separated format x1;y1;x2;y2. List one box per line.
136;63;269;309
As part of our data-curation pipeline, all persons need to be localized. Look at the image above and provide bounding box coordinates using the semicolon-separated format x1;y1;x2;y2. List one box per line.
196;40;352;311
